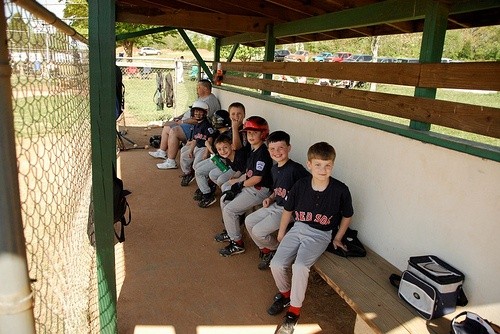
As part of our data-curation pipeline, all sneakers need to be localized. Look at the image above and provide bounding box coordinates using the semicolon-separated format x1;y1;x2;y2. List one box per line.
180;174;196;185
149;149;167;158
268;293;290;314
156;159;178;168
275;311;300;334
258;248;277;268
215;229;231;241
193;189;203;200
198;195;217;208
219;241;246;256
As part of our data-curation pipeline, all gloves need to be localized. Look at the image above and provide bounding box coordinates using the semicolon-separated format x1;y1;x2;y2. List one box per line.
231;181;244;193
220;190;235;202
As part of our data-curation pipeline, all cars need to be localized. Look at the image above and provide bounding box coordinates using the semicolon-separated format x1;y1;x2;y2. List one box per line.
274;50;289;62
326;52;351;62
344;55;372;61
138;47;163;55
371;57;452;63
284;51;310;62
312;52;332;62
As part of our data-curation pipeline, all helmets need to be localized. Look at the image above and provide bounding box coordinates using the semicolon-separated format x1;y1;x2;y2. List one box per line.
239;116;269;141
452;312;497;334
191;102;208;116
214;110;230;129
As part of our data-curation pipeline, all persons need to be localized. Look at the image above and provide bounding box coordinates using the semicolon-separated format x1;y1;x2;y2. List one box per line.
244;131;313;270
266;142;354;334
176;55;184;83
149;79;221;169
193;101;246;208
180;101;215;186
214;116;270;258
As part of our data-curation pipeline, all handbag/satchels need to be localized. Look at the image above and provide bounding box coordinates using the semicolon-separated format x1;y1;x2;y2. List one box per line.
113;163;132;242
150;135;161;147
327;223;366;257
389;255;468;320
116;64;125;119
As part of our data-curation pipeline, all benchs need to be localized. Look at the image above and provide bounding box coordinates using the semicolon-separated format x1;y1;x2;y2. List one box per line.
251;203;453;334
